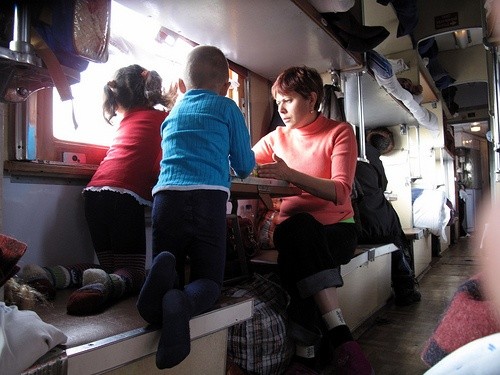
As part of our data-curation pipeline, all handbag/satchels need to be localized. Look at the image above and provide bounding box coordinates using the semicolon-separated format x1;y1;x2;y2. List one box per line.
227;275;295;374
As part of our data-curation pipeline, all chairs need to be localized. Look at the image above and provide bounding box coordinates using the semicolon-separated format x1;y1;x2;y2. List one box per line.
0;156;433;375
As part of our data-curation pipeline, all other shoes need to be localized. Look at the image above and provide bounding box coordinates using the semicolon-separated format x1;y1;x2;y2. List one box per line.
339;342;374;375
393;283;421;301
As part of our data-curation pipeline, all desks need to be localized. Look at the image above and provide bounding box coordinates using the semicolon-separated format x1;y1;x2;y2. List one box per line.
3;161;302;211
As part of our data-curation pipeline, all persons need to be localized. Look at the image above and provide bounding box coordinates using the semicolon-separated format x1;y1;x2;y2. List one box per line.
422;190;500;375
24;64;178;301
135;46;255;369
353;126;422;305
250;66;357;375
